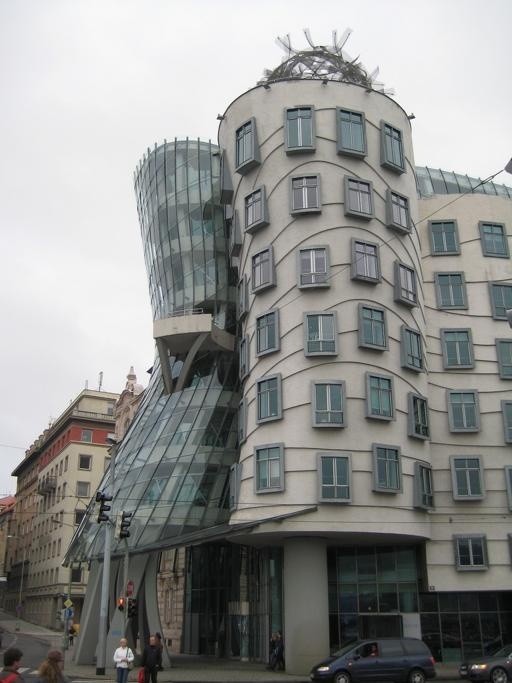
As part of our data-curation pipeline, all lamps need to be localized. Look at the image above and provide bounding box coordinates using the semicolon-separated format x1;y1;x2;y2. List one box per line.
118;597;124;611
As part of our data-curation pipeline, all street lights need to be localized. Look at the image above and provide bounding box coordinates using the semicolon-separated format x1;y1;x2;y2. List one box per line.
52;519;77;650
7;534;26;631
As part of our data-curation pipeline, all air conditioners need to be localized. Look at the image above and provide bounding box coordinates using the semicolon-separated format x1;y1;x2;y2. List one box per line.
37;475;56;497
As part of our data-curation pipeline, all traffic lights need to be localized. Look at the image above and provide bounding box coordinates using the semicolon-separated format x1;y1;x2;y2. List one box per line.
118;596;125;611
120;510;132;538
98;493;113;523
126;597;137;618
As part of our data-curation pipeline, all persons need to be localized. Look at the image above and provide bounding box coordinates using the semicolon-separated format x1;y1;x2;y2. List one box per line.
265;630;283;670
269;633;285;669
0;646;27;682
39;650;67;683
140;634;163;682
368;644;378;656
113;637;135;683
68;625;76;645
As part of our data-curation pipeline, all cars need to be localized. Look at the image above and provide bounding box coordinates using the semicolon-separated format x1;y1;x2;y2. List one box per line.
458;643;512;683
309;636;436;682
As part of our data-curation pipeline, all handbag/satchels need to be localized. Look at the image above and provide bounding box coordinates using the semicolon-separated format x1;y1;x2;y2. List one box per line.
138;667;145;683
128;662;134;671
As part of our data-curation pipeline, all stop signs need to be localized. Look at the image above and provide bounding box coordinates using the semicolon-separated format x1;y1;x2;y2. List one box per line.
127;581;134;594
